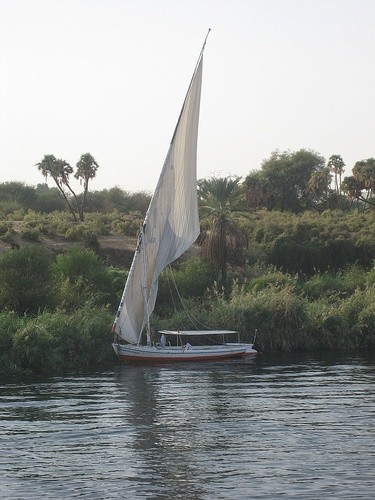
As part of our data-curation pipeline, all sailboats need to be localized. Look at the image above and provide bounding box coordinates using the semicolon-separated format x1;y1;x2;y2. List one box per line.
109;28;261;361
